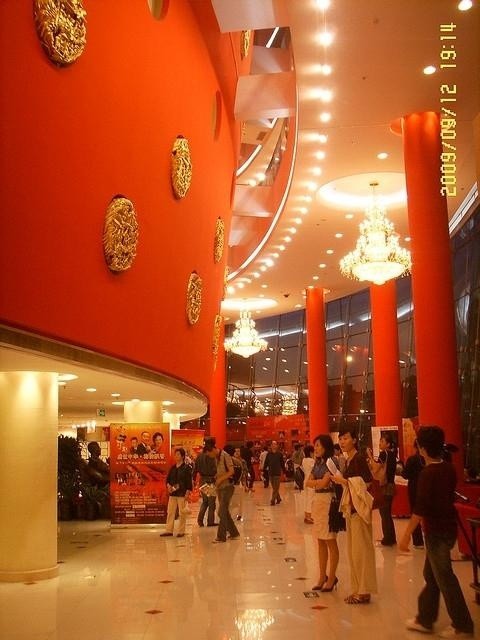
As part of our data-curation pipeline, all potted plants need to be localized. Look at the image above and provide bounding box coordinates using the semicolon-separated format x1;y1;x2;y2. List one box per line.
58;431;110;520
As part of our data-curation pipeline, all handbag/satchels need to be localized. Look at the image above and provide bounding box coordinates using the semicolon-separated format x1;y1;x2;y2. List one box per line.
382;481;398;495
328;488;347;531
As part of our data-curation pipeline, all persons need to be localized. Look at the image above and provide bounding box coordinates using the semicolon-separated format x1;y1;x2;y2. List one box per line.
330;426;377;603
114;430;164;454
301;436;424;552
159;449;192;537
203;445;241;542
115;464;145;485
306;435;339;592
86;440;110;490
399;426;475;639
225;441;305;506
191;438;218;526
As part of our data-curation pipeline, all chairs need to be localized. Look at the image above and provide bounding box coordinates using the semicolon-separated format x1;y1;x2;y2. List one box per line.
369;475;480;565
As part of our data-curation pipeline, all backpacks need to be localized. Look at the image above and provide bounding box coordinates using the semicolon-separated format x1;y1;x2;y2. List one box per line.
222;452;243;484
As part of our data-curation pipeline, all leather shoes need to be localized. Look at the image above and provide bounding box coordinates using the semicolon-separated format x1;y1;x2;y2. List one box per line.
344;593;371;605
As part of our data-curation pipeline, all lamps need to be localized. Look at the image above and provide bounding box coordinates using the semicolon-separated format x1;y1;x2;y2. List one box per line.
224;305;269;358
337;179;411;288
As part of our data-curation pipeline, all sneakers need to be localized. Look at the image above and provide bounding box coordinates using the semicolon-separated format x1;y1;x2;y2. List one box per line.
211;538;227;544
160;532;186;538
199;521;219;527
439;625;477;640
269;498;282;506
303;518;314;525
228;534;242;541
406;616;434;635
375;537;425;553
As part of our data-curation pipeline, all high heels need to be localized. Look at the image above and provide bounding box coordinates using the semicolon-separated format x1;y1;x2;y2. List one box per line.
312;573;328;591
320;576;339;593
